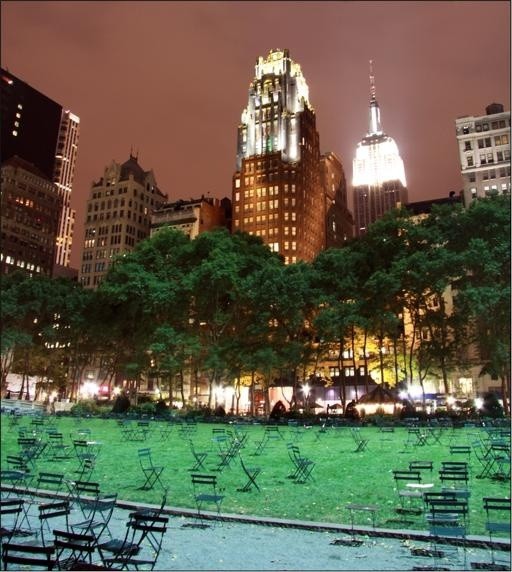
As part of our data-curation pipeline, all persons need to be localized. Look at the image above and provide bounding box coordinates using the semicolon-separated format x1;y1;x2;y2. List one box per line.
112;391;130;413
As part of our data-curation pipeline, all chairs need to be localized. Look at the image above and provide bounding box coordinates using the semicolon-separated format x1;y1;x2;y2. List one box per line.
0;414;512;572
